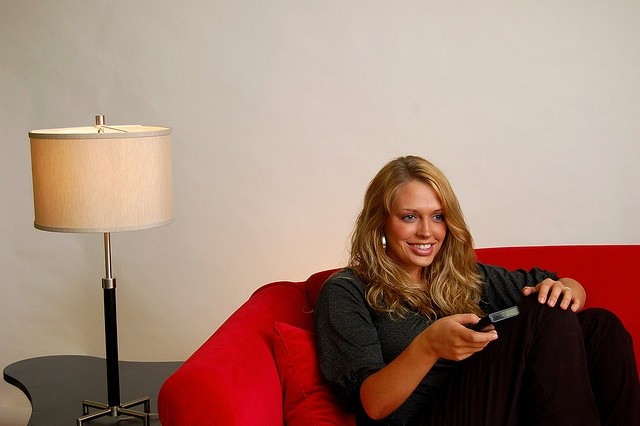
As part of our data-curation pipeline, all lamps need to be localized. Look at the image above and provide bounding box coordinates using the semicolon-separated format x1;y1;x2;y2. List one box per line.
28;112;173;425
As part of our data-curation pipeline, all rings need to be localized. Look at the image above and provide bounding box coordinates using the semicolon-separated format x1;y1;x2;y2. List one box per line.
562;287;572;291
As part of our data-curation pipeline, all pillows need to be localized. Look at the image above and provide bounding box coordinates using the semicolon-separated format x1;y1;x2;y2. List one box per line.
269;318;353;425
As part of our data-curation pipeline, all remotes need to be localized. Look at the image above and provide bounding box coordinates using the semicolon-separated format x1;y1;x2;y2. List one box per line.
475;305;520;330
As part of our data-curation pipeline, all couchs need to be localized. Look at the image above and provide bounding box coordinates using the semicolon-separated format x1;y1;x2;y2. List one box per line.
159;244;639;424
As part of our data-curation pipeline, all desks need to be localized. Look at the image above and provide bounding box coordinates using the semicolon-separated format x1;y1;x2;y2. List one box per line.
2;353;184;425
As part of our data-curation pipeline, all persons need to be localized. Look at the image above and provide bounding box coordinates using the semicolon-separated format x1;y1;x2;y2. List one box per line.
313;156;640;426
